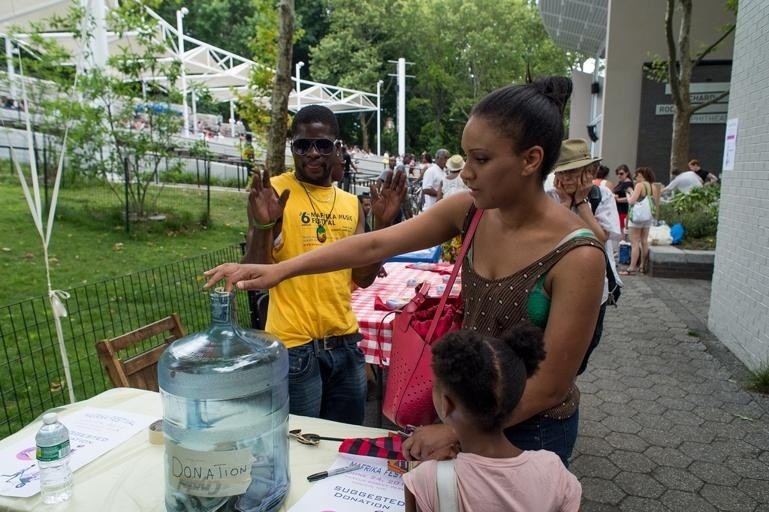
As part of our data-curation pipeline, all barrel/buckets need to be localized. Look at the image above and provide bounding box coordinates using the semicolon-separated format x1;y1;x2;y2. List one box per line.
156;290;291;511
618;241;631;265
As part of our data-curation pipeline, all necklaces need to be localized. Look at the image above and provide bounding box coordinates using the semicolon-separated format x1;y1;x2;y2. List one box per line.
294;174;337;243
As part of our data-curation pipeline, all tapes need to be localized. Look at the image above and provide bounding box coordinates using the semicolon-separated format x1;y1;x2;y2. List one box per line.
148;420;165;445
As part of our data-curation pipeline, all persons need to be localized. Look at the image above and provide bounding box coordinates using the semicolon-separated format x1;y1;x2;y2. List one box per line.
241;105;408;427
545;138;722;383
200;75;607;471
401;320;582;512
332;146;472;232
244;134;255;183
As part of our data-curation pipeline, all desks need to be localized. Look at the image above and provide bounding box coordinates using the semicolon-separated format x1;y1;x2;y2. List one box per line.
351;262;461;428
0;386;406;512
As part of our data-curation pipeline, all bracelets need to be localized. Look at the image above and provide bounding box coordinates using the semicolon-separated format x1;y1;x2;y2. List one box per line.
252;218;277;230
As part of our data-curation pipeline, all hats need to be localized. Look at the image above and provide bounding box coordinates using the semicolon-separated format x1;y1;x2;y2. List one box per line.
445;153;465;172
554;138;602;173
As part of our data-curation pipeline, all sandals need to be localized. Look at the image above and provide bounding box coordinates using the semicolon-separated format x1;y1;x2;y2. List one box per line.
619;267;647;275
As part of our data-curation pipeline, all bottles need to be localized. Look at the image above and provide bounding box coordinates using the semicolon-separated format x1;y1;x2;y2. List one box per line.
34;411;76;505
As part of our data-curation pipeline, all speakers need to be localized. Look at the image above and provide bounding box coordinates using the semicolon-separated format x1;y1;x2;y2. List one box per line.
591;83;599;94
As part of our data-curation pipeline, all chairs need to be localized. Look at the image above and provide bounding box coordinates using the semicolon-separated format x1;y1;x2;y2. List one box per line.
96;312;186;392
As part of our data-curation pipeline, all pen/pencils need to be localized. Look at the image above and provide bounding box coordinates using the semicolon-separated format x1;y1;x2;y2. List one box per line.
307;464;360;482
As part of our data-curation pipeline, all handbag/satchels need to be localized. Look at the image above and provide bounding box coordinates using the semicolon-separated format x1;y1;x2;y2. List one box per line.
378;209;483;428
631;197;653;223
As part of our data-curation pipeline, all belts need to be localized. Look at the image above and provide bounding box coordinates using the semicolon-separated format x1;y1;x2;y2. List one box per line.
309;332;365;350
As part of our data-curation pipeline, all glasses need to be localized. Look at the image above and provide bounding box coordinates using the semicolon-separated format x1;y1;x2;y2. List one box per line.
291;137;338;156
616;173;625;176
